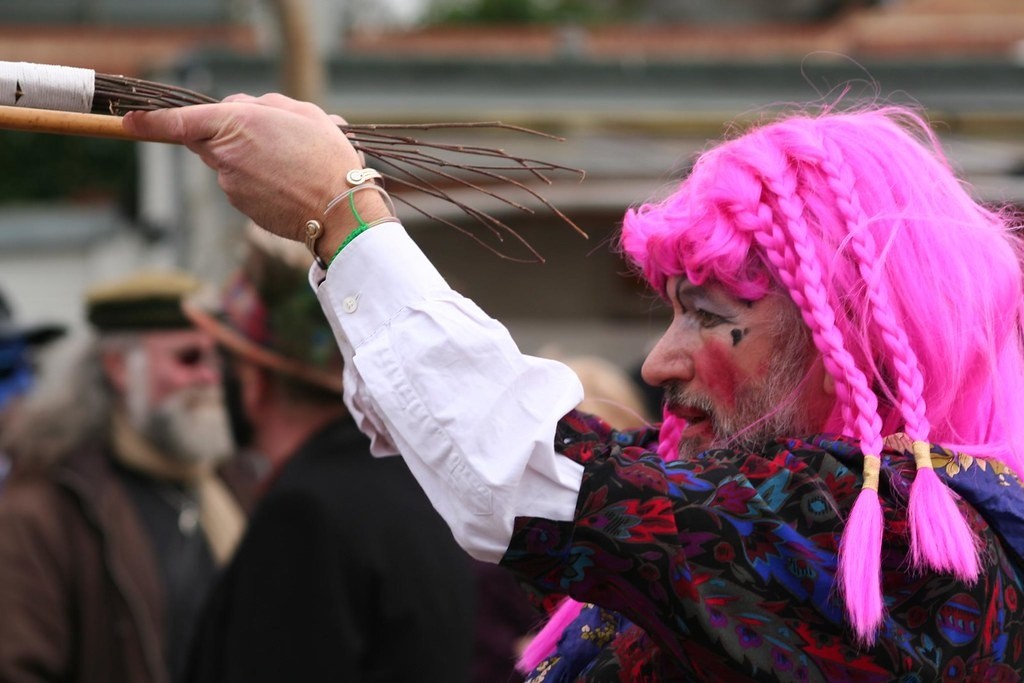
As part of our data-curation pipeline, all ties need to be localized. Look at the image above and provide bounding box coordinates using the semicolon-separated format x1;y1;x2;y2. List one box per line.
185;458;249;565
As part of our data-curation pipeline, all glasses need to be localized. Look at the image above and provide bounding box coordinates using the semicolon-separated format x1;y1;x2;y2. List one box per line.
179;343;202;366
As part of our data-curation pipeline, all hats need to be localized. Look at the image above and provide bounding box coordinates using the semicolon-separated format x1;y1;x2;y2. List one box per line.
182;215;342;394
87;277;197;334
0;292;67;349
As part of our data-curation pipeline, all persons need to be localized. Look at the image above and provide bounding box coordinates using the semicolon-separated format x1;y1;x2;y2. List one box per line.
2;211;682;683
116;88;1023;682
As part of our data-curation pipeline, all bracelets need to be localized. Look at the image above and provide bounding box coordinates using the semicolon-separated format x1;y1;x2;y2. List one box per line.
304;160;402;273
